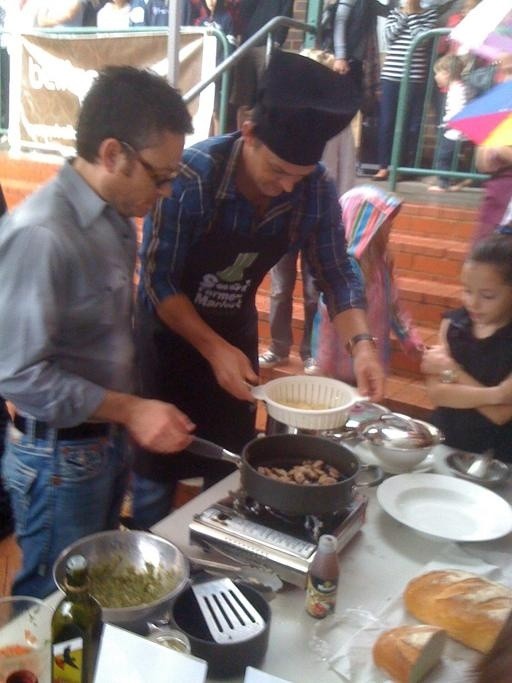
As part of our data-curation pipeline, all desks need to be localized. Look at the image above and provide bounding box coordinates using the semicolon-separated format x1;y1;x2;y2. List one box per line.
0;419;512;683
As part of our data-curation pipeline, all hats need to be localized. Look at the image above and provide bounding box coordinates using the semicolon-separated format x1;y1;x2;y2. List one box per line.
250;47;358;165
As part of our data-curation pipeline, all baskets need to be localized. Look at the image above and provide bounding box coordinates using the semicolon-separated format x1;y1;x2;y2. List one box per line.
250;374;371;430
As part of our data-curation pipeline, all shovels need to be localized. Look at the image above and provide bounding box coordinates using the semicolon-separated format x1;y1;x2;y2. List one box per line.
188;559;266;645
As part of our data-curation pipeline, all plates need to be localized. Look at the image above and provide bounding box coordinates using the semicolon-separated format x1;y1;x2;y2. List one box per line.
377;473;512;542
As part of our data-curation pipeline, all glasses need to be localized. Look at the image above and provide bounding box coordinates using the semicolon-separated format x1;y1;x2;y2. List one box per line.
95;136;179;186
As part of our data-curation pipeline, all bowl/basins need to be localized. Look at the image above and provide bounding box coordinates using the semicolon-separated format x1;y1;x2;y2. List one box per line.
171;583;272;675
367;443;432;471
446;453;509;484
252;375;372;431
53;531;188;626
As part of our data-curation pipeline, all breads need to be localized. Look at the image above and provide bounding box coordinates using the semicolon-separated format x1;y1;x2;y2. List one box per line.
402;570;512;654
373;624;446;683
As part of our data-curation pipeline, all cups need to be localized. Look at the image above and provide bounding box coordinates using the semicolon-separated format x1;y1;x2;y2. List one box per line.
142;620;190;655
0;595;51;683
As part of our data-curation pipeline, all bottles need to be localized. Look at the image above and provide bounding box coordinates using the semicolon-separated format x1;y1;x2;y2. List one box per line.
51;555;104;682
303;535;341;621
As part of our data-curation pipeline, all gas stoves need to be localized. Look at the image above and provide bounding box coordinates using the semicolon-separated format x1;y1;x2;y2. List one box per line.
189;487;371;589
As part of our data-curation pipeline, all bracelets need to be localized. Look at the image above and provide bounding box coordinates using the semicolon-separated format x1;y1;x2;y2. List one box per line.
439;365;466;385
345;333;379;359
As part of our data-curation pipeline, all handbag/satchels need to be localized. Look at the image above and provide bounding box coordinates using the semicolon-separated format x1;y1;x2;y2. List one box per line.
319;0;339;51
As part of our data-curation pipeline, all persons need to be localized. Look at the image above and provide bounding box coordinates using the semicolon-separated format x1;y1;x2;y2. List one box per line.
2;63;197;616
260;47;357;373
0;0;512;192
468;113;512;249
128;52;386;529
309;184;428;387
418;232;512;463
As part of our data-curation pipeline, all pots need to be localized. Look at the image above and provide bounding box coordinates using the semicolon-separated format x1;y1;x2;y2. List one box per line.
187;434;361;513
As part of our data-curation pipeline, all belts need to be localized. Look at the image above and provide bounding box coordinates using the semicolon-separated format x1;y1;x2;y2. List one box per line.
14;409;109;442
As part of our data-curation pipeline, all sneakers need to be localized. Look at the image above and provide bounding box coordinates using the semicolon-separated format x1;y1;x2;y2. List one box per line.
304;357;321;376
257;351;288;368
371;173;386;181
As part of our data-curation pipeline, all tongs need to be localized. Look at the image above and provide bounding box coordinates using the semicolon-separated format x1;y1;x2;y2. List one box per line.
190;556;288;597
